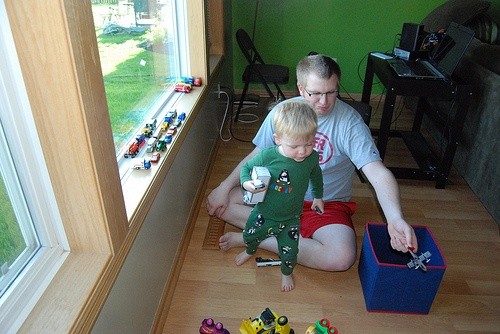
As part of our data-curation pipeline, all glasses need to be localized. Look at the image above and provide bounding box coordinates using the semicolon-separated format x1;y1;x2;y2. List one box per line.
301;84;338;98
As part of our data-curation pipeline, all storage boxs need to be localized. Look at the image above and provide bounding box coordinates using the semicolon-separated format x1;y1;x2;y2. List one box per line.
358;223;447;315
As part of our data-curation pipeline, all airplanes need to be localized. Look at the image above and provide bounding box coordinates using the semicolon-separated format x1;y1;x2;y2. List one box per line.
406;247;433;273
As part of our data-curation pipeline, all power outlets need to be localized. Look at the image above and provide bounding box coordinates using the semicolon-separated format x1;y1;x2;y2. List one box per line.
218;80;222;99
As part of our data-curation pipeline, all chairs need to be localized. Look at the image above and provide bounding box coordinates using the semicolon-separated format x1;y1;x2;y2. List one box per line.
234;28;290;124
308;51;373;184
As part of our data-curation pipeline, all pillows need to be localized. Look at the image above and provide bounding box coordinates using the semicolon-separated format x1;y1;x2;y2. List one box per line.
420;0;492;35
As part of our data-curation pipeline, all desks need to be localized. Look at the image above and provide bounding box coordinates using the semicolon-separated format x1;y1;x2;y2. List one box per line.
359;51;472;189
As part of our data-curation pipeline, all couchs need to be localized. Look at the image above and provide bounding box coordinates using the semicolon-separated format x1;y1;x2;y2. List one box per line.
402;14;500;226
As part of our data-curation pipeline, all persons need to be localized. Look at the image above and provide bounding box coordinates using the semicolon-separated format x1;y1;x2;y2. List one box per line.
206;55;418;272
234;101;324;292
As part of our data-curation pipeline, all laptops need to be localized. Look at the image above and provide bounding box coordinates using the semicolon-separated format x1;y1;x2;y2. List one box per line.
386;22;475;79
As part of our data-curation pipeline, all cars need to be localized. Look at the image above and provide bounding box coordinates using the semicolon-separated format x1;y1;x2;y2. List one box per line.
199;317;231;334
239;307;295;334
174;76;201;93
123;109;186;170
304;318;339;334
